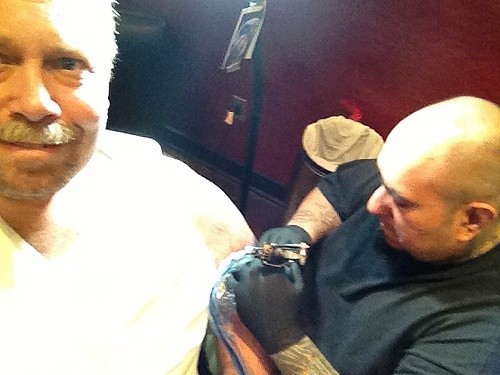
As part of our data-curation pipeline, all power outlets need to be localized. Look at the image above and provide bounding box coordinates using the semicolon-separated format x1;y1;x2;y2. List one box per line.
232;95;247;121
171;75;193;100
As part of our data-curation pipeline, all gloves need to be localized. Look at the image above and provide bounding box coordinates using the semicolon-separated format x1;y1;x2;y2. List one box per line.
258;224;313;256
235;268;306;355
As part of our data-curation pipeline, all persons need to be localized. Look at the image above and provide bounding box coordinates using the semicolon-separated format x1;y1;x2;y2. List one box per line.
0;1;259;375
199;96;500;374
